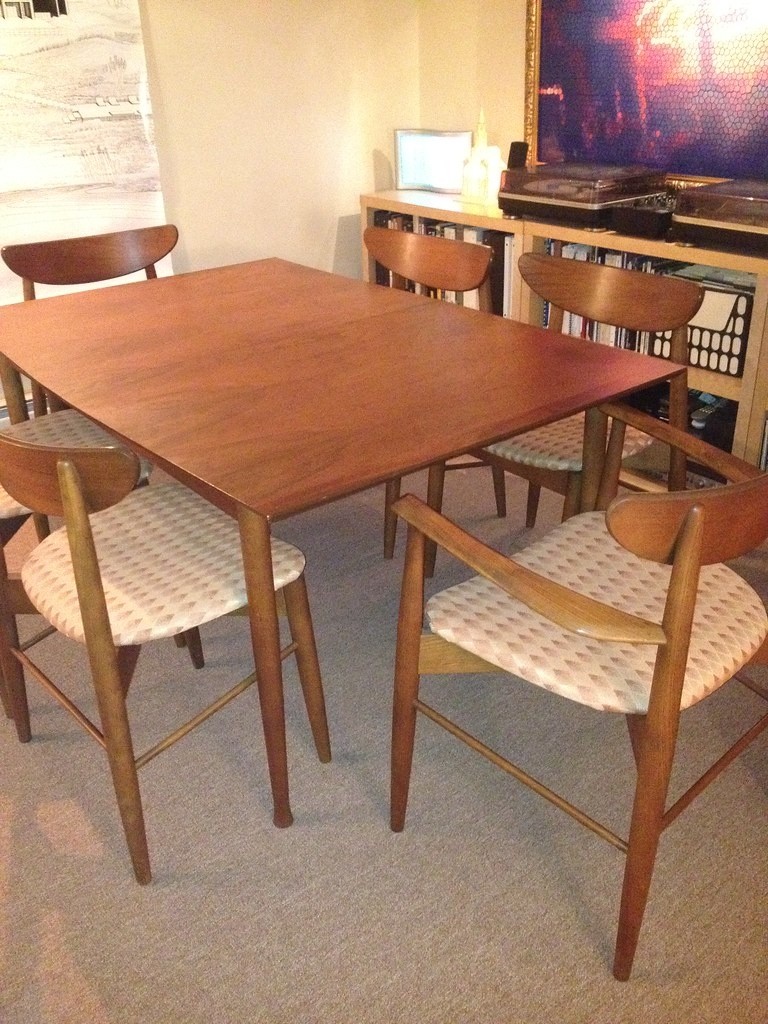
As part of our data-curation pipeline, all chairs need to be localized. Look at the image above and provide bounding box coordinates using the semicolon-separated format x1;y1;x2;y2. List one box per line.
0;225;768;982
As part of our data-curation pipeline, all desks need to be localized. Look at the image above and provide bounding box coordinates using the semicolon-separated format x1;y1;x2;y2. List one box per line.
5;256;687;830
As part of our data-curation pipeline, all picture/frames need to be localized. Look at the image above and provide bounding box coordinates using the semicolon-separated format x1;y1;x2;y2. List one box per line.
523;0;768;185
393;128;476;195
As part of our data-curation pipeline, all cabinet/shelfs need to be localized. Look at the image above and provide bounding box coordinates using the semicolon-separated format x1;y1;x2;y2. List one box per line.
361;186;768;488
